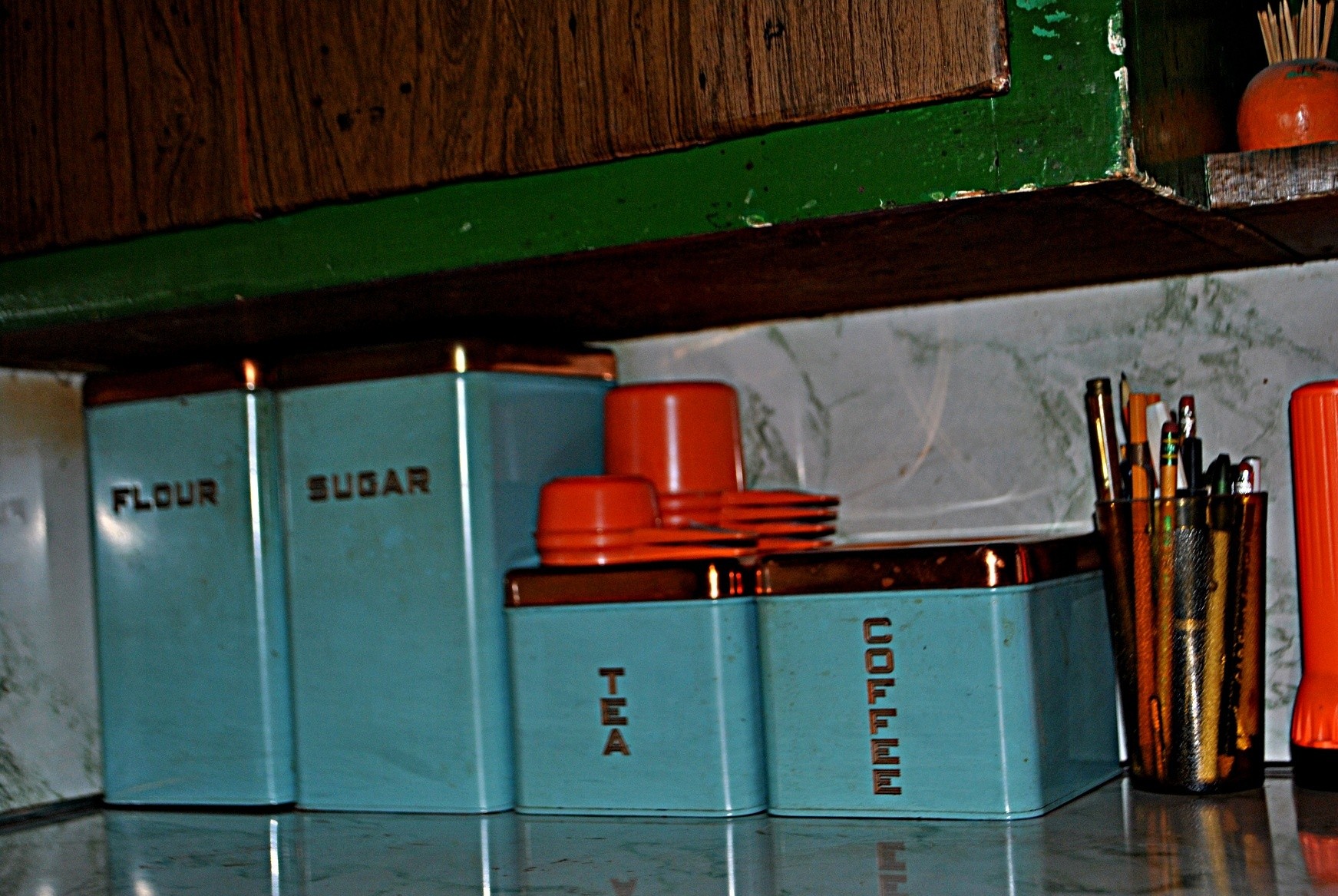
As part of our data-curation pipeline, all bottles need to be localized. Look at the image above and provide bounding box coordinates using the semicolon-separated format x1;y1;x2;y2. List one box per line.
1238;59;1338;151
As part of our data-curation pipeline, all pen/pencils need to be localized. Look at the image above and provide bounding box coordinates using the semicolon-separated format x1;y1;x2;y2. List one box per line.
1084;371;1263;786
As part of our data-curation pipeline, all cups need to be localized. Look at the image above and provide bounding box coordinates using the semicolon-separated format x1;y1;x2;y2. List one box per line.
1093;492;1267;796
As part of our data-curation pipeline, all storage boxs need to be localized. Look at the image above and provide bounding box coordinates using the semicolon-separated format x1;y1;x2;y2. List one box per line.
80;344;1125;820
